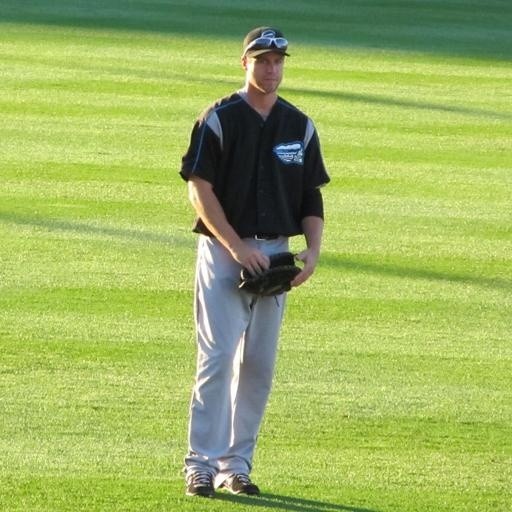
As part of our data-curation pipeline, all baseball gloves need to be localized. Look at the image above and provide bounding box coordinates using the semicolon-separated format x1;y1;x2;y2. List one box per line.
237;252;302;296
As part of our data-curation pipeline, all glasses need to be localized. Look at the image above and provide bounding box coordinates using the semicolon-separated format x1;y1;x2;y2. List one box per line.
241;37;288;58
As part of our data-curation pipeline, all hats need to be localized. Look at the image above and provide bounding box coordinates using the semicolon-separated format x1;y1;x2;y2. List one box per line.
242;27;288;59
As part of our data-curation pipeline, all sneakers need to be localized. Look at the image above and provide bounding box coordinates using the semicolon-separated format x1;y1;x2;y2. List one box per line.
217;467;260;496
184;465;215;499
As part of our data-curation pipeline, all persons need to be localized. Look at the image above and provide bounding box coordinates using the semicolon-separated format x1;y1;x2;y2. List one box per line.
178;25;331;497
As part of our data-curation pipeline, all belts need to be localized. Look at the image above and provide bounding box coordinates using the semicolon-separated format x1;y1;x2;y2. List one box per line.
241;232;278;242
